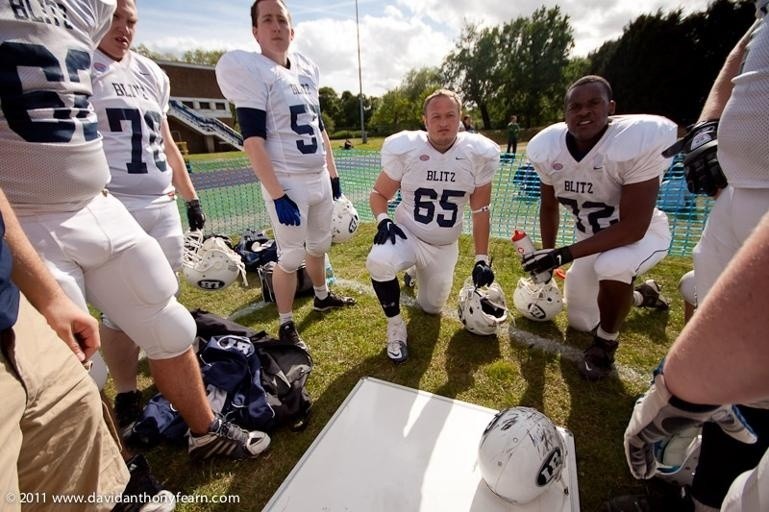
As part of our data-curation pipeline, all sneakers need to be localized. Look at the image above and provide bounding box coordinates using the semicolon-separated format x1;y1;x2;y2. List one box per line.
635;279;668;310
313;289;358;312
387;320;408;362
188;417;271;458
279;321;308;352
115;389;150;447
122;452;176;512
584;322;618;381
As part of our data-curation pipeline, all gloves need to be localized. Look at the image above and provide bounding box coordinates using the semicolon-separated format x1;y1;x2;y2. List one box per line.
185;200;205;231
472;261;494;288
521;246;573;285
274;194;300;226
330;177;341;201
624;353;757;479
374;219;407;245
662;119;728;196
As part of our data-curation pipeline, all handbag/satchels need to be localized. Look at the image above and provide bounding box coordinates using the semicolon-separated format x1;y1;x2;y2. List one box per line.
257;253;337;303
132;334;275;443
234;229;278;271
191;308;313;432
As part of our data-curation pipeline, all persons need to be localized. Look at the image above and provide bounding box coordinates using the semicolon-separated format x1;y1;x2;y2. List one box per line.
0;190;132;511
457;113;475;133
366;90;496;363
662;1;769;323
1;0;271;511
345;137;353;150
217;0;357;350
520;75;678;382
624;209;769;511
89;0;207;451
507;114;520;153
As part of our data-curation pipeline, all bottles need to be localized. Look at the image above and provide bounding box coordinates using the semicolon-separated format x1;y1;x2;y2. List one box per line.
325;252;336;284
512;227;553;285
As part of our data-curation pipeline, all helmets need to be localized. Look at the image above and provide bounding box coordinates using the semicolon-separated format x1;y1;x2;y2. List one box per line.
331;193;359;244
513;277;562;322
458;276;509;335
479;406;568;505
181;227;249;291
